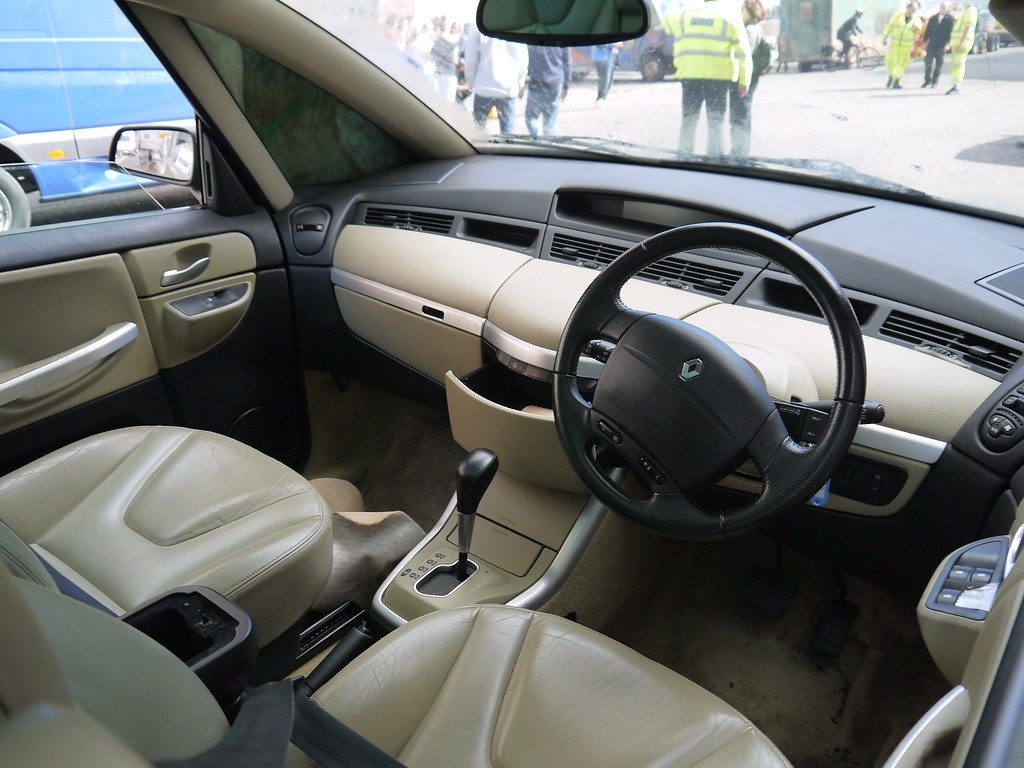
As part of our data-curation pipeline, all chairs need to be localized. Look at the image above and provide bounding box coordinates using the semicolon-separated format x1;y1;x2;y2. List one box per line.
0;424;334;665
0;599;791;768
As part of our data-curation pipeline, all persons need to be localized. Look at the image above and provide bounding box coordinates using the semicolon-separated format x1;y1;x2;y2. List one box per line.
946;0;977;95
882;1;923;89
385;13;472;102
836;7;867;62
525;46;572;136
660;0;753;155
775;36;787;73
466;26;529;135
592;41;623;107
922;2;956;88
729;0;767;153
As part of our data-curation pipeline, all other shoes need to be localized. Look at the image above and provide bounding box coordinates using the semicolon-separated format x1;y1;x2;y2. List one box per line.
921;81;937;88
946;88;958;95
887;78;902;89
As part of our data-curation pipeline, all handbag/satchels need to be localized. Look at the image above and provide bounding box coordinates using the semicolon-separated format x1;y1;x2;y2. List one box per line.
752;39;771;79
